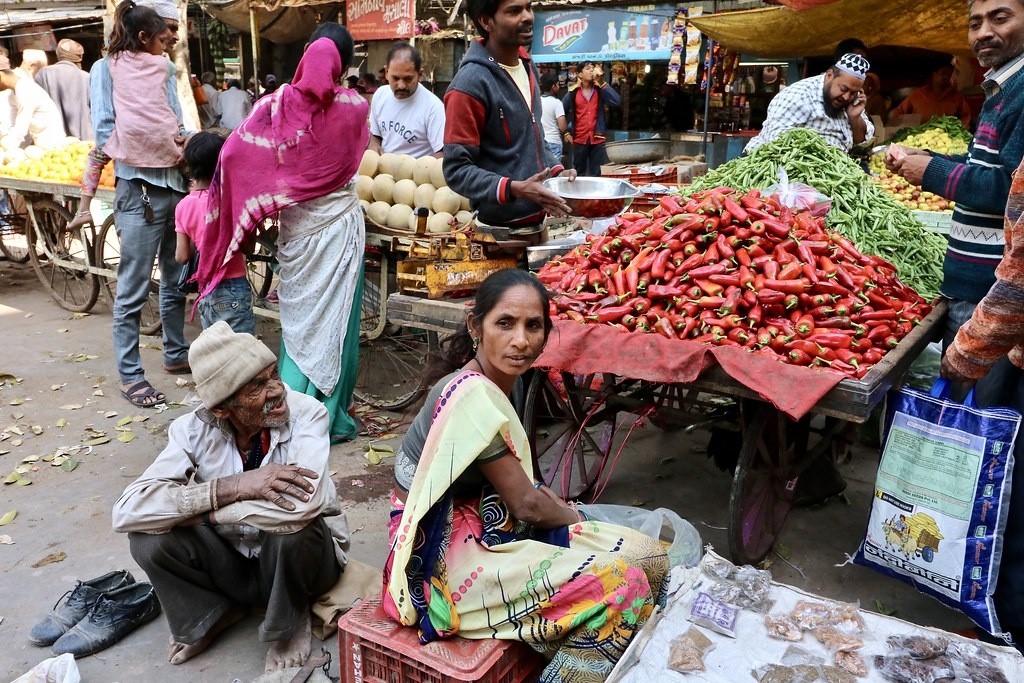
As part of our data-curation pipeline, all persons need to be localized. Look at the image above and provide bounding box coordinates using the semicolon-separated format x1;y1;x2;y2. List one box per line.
199;24;371;439
381;268;670;683
366;43;445;159
176;131;255;335
883;1;1024;411
936;157;1024;649
833;39;972;131
198;72;280;131
742;53;878;157
563;62;621;176
0;39;94;235
540;73;567;162
443;0;577;242
650;79;693;132
64;0;190;407
111;320;351;676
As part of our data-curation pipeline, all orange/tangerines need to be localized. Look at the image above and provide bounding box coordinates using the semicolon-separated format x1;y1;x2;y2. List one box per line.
74;158;116;189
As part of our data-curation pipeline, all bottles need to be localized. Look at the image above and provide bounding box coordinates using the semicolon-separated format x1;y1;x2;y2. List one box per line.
608;15;672;53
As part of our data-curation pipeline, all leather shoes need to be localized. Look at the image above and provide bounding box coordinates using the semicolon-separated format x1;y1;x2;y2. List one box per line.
27;568;136;645
51;581;161;659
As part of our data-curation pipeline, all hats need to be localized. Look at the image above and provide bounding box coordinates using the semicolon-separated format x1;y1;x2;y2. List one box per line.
128;0;180;21
55;38;84;62
22;49;48;62
187;320;276;412
0;54;10;70
760;64;780;85
344;66;360;81
834;53;871;80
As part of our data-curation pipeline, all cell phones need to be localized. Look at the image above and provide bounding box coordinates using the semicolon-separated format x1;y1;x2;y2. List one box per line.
853;91;861;106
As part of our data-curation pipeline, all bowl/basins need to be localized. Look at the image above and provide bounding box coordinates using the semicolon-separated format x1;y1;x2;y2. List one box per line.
542;176;645;221
604;137;672;164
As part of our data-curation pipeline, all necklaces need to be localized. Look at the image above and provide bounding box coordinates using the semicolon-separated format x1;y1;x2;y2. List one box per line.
255;441;262;468
474;358;485;376
195;189;207;197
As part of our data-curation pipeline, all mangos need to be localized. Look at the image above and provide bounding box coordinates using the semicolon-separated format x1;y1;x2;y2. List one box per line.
879;171;956;214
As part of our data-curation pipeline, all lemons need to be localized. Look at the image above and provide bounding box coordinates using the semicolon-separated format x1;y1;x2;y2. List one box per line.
0;143;93;185
869;128;969;174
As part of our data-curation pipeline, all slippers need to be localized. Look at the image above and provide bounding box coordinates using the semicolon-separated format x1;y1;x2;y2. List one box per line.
169;358;192;375
120;381;168;407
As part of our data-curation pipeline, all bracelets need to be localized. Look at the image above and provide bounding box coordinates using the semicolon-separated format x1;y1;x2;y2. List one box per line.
202;511;217;526
535;482;544;489
213;478;219;510
578;510;590;522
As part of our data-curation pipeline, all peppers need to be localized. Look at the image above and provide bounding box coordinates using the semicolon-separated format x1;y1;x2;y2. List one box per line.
872;114;973;144
450;186;936;380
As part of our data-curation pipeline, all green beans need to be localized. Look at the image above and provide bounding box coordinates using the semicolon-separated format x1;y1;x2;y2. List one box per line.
674;126;951;303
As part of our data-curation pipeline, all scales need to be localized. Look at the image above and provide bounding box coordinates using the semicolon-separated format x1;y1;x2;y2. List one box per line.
359;204;531;300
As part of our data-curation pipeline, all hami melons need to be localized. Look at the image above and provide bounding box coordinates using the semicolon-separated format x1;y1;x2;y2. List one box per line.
356;149;474;234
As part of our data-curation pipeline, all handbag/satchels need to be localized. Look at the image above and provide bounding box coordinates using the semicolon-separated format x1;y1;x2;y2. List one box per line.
759;165;832;218
849;376;1022;645
568;497;703;575
176;252;199;294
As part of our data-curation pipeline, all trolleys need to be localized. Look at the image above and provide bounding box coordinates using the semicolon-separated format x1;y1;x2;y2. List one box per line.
0;177;944;570
